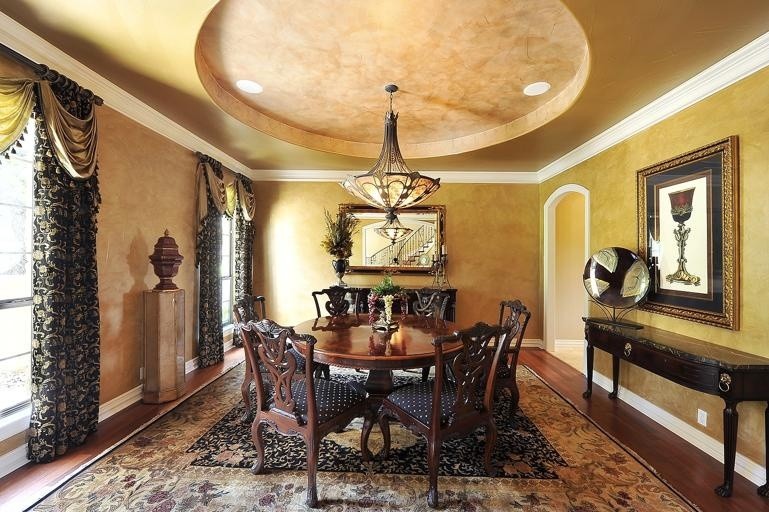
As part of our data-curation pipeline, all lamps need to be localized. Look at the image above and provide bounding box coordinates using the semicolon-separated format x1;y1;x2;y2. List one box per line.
337;84;441;224
373;215;413;245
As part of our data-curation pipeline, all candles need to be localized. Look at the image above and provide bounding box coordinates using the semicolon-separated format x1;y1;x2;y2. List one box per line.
441;245;447;255
433;254;439;261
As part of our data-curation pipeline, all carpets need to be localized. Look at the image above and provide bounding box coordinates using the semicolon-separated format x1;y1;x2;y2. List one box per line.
23;363;702;512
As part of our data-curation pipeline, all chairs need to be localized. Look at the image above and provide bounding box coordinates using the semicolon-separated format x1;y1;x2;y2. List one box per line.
237;319;368;507
442;298;531;430
312;286;360;377
374;323;511;508
411;293;454;382
231;294;330;420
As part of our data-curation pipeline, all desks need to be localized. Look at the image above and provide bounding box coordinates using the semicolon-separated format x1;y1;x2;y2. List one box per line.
582;317;769;497
290;314;464;422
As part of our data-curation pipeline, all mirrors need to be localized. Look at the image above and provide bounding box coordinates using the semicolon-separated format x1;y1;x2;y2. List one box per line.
583;246;652;309
337;203;446;275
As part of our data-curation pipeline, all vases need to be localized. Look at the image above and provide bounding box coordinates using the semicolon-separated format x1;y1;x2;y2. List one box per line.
331;259;348;288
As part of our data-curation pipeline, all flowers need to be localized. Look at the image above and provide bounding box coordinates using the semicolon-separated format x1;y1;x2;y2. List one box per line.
320;207;359;260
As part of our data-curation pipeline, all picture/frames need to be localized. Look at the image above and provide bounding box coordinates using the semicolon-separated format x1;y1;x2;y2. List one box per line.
636;134;741;332
